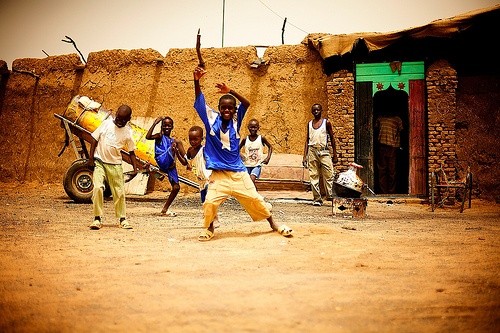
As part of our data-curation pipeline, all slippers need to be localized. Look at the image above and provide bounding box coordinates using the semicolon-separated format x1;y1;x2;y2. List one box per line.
119;219;133;228
198;229;215;241
273;224;294;237
90;220;102;229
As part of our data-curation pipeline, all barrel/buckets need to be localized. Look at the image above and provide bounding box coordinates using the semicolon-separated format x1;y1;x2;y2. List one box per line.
63;94;163;167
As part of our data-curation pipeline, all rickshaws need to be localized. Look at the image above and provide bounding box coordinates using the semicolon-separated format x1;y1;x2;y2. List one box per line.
53;94;201;205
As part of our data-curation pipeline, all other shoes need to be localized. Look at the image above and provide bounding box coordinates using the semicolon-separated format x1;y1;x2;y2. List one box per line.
213;217;220;228
313;200;323;207
159;210;177;217
326;196;334;201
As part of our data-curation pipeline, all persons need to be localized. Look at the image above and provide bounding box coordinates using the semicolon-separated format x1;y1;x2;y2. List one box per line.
88;105;138;230
171;126;220;229
302;103;338;206
145;114;187;217
375;116;403;193
239;119;273;194
190;67;295;241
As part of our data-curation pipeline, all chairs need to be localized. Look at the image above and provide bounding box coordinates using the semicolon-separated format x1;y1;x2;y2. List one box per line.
431;159;473;213
